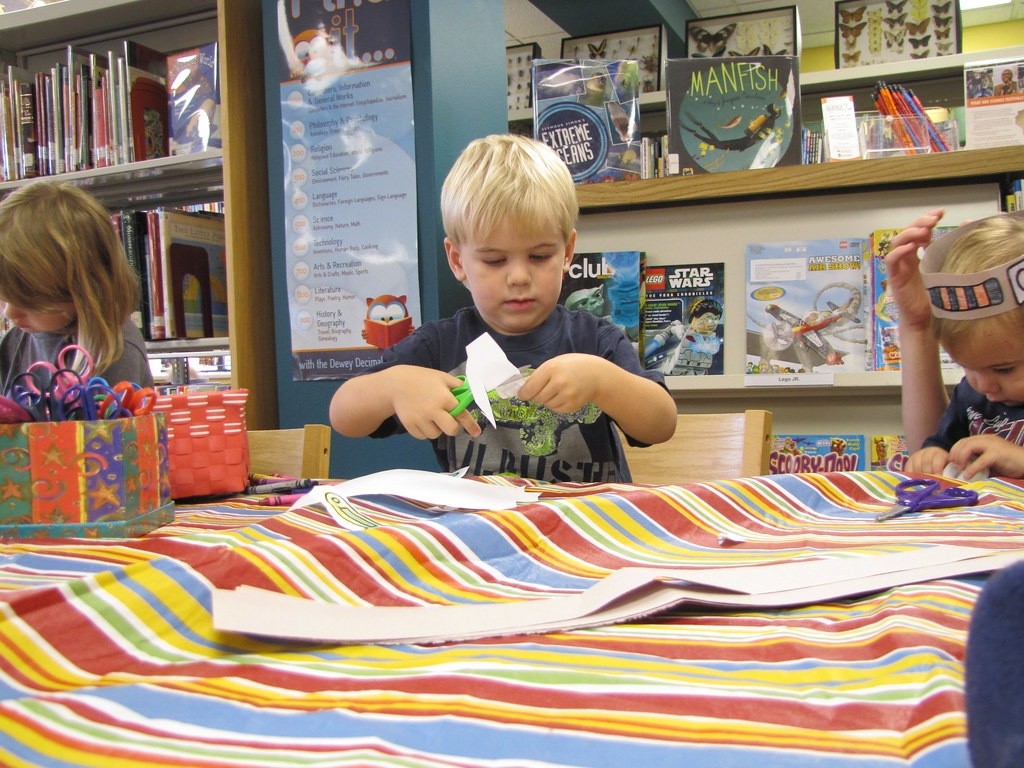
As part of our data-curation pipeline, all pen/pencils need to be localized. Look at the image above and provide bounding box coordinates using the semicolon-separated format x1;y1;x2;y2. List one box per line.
870;80;951;155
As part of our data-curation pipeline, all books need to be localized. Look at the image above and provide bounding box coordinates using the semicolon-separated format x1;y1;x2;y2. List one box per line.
802;128;822;164
0;41;225;182
640;135;669;179
107;209;228;337
155;382;230;396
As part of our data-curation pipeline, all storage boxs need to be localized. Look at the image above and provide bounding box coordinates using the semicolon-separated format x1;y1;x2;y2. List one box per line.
0;412;175;538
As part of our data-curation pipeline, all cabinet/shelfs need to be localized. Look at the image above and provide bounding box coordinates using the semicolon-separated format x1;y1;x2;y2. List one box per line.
0;0;279;433
573;143;1024;400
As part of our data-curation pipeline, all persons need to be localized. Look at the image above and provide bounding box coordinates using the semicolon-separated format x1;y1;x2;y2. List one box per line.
994;69;1016;96
883;209;950;454
903;210;1024;478
329;133;677;482
0;178;153;402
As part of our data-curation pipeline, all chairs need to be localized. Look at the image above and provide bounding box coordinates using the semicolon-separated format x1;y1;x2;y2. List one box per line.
244;420;332;478
611;408;770;482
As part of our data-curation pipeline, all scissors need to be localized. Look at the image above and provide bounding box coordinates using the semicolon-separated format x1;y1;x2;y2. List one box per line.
446;364;532;418
12;344;157;420
876;479;978;521
58;386;119;420
